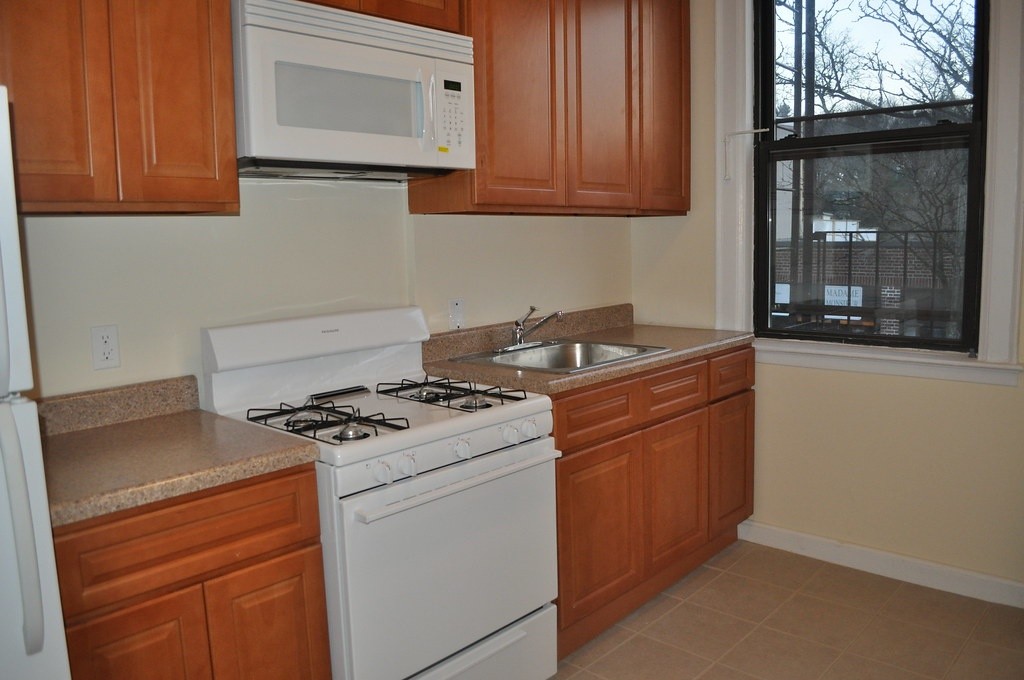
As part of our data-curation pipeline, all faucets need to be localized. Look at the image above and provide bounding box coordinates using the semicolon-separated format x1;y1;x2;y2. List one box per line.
512;306;564;346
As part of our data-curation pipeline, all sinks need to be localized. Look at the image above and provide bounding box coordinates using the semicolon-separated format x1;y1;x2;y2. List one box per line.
448;339;673;375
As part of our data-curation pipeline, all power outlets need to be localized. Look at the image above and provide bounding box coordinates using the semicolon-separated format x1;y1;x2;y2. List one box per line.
91;325;121;371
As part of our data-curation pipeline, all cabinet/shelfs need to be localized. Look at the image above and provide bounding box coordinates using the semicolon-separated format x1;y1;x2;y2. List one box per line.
0;0;241;217
549;342;754;660
52;462;333;680
322;0;691;217
